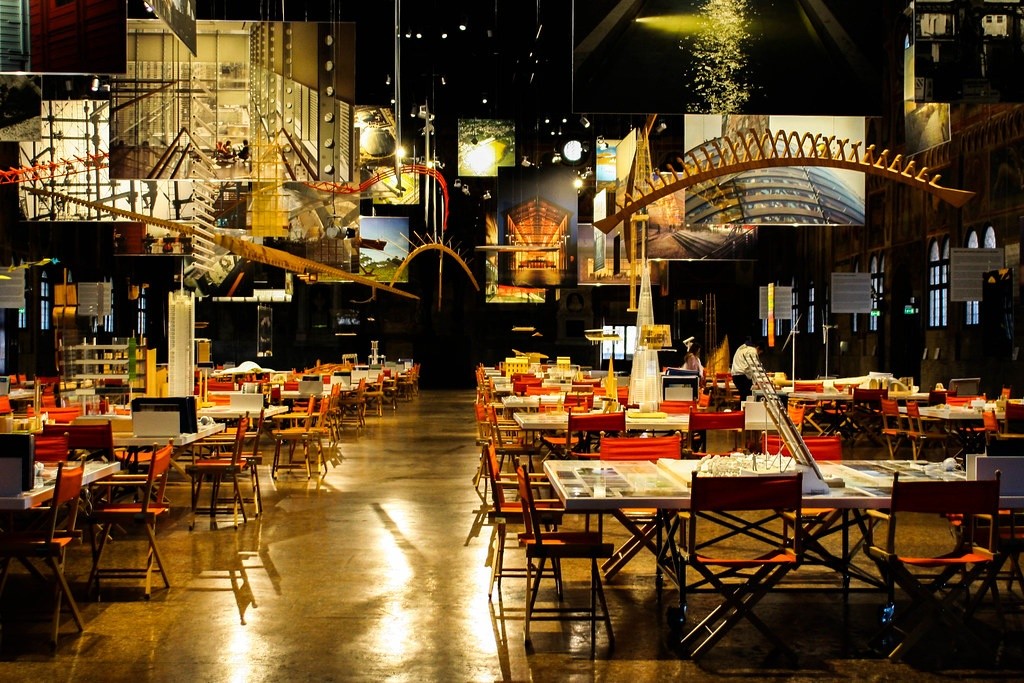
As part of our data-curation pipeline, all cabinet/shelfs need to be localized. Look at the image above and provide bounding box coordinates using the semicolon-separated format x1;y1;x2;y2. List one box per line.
61;344;156;396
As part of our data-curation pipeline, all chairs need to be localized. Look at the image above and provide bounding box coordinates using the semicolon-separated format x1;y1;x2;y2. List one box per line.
0;363;1023;665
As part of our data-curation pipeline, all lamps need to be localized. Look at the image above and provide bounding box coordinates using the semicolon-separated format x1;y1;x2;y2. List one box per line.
382;0;669;201
65;75;110;93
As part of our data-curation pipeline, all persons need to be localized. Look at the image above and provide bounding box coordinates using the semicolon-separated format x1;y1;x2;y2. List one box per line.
731;340;764;409
685;344;701;371
218;140;248;167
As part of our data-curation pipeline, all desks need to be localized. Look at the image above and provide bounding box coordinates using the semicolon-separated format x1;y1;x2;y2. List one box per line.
0;461;122;603
540;454;1024;629
110;422;226;504
503;395;563;413
513;411;779;454
706;383;736;410
785;392;931;435
280;392;331;426
194;405;287;455
897;405;1005;456
